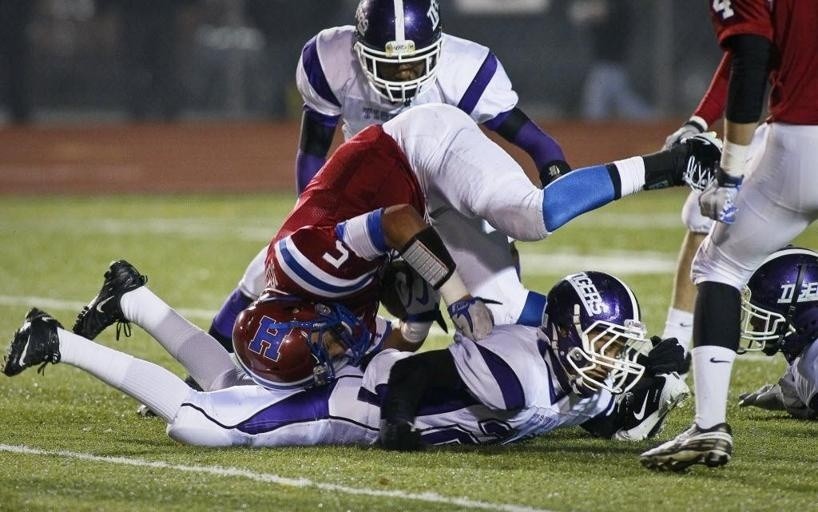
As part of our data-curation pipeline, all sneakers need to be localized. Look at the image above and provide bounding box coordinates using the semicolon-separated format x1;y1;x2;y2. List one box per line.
681;130;723;193
70;258;148;340
639;422;733;474
611;370;691;441
0;307;64;377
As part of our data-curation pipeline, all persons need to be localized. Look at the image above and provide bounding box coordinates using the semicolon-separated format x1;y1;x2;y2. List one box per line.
637;1;815;471
136;1;575;422
614;39;770;447
4;259;688;450
231;102;728;444
732;243;817;460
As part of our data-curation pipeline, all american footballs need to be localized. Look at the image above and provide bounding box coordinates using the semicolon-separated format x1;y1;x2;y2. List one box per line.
382;258;421;319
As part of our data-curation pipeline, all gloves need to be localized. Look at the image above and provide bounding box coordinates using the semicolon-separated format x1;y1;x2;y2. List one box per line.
738;384;785;410
380;419;425;451
779;365;809;416
699;166;744;221
397;260;441;322
649;335;684;375
665;120;704;150
447;296;494;345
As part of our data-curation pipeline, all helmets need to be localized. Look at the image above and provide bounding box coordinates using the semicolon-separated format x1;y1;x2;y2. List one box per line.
232;295;370;393
737;246;818;356
352;0;442;105
537;270;649;400
